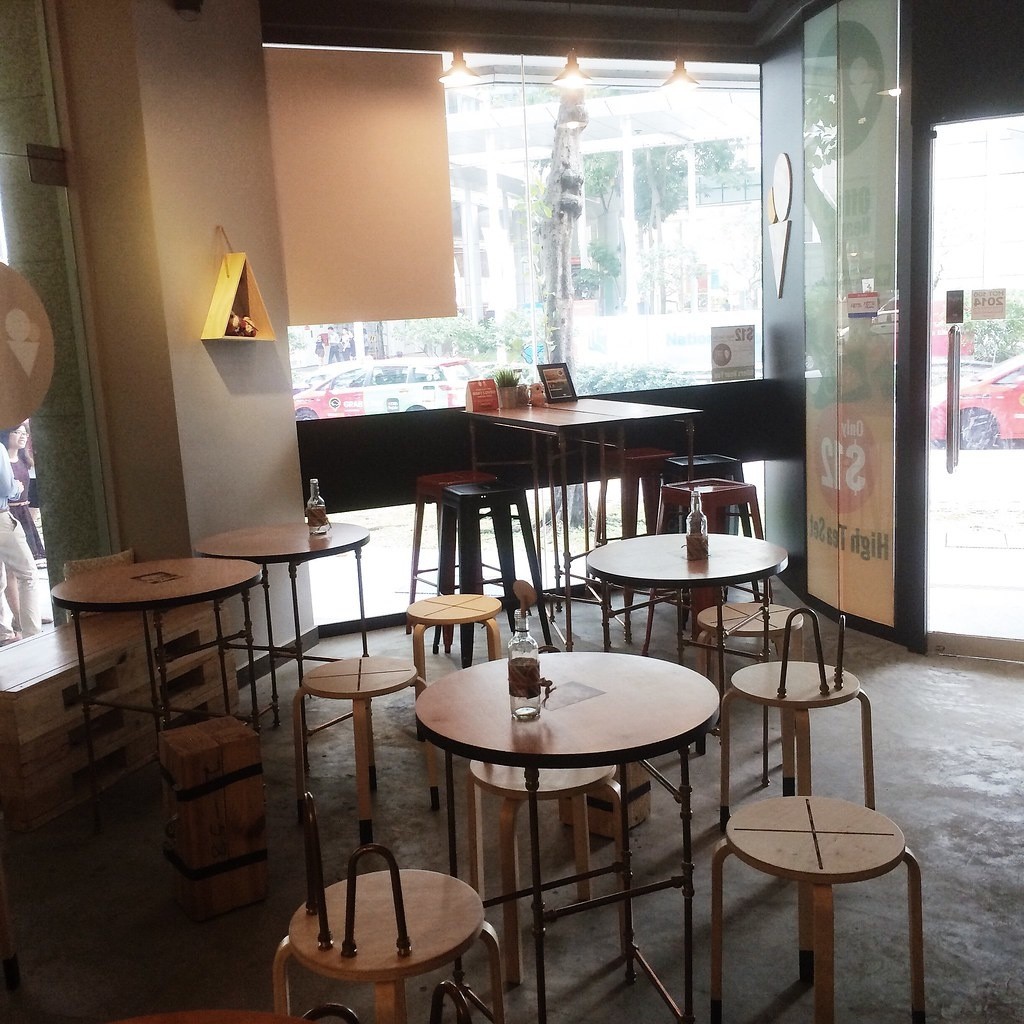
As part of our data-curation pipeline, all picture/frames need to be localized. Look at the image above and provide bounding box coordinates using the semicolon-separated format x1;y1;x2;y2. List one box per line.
536;363;578;405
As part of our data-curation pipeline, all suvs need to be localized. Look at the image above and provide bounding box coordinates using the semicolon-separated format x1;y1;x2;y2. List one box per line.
293;354;485;423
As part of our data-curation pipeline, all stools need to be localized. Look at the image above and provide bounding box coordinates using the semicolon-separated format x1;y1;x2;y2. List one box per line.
466;761;626;983
720;607;875;833
272;793;505;1024
409;470;498;654
157;716;269;922
406;594;501;741
711;797;926;1024
558;759;651;837
432;479;554;669
695;603;804;736
642;477;773;672
294;656;441;847
590;447;676;605
665;455;761;630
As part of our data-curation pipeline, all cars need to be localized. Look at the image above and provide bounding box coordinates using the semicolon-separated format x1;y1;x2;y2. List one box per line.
288;359;373;394
927;351;1024;451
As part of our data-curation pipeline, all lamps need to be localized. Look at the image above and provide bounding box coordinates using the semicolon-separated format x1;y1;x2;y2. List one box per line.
438;0;700;87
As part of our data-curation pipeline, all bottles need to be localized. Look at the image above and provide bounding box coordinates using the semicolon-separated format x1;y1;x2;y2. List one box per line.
306;478;327;536
685;490;709;561
507;608;541;722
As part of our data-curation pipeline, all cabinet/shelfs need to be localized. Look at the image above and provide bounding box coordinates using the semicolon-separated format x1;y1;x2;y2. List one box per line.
0;597;240;837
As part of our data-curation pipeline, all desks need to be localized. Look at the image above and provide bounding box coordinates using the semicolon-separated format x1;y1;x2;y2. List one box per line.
192;523;370;769
51;557;263;827
462;399;703;653
585;533;788;789
414;651;720;1024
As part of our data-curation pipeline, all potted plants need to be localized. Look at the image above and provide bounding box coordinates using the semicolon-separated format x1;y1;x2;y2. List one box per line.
492;369;521;409
